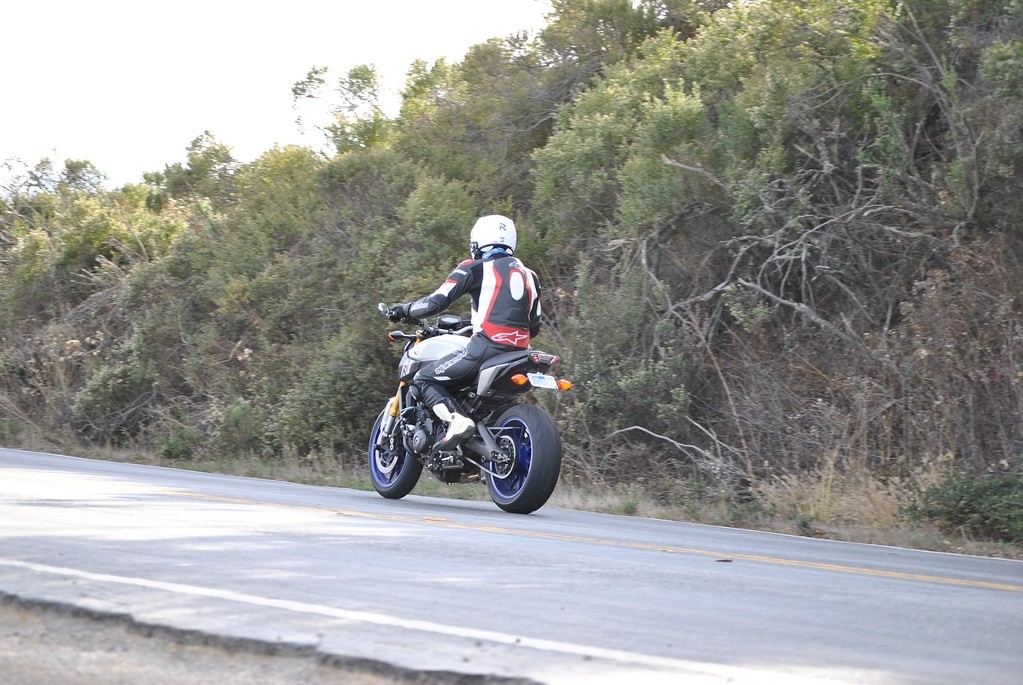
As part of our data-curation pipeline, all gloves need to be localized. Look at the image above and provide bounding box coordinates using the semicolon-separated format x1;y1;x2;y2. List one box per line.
389;303;412;324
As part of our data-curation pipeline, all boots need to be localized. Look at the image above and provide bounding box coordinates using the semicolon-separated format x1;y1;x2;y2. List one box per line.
429;397;475;454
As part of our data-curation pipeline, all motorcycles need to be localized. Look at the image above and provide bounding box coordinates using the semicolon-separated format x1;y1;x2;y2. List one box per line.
366;302;574;515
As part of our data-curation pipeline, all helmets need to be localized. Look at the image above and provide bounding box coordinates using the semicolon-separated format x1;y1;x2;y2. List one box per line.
470;215;518;261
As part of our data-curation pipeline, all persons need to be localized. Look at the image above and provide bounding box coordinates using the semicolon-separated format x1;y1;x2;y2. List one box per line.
391;214;543;452
